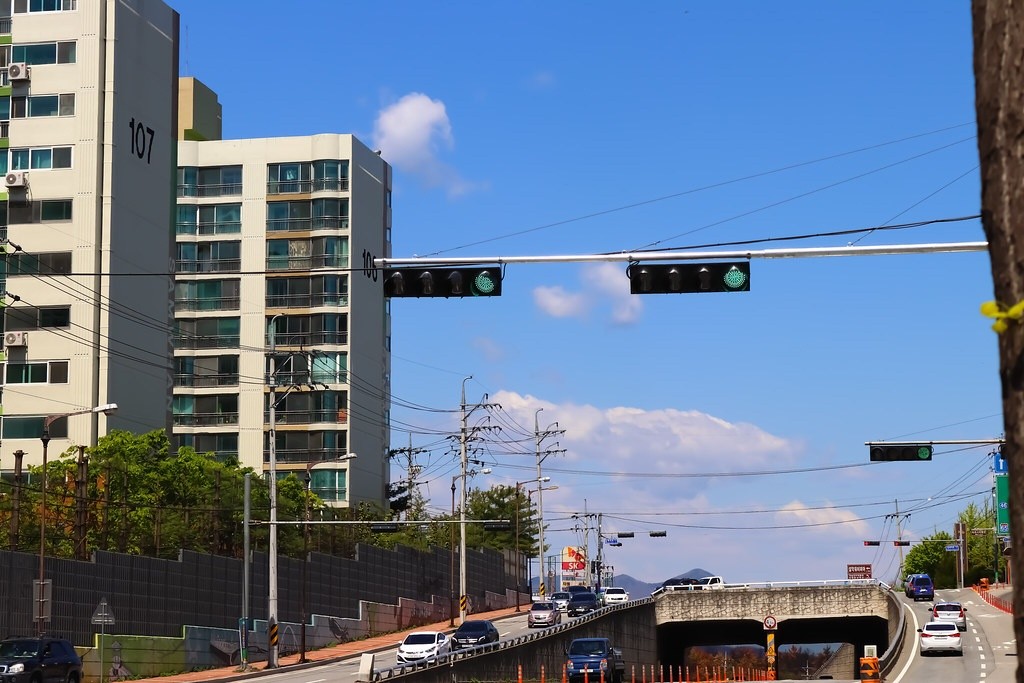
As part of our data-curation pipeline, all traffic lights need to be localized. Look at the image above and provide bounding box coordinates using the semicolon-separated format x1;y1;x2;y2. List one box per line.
869;444;933;461
628;260;754;296
864;540;880;547
894;541;910;546
382;264;504;299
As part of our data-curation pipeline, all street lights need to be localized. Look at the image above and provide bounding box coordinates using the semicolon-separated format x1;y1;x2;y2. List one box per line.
296;450;359;666
449;467;493;626
515;475;552;612
529;484;559;602
38;402;121;641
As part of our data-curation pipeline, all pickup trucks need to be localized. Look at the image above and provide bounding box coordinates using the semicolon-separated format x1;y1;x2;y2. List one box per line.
564;638;627;682
698;576;750;589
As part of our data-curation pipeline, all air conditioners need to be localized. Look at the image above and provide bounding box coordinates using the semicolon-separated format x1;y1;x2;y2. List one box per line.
5;173;25;188
8;62;29;80
4;330;27;345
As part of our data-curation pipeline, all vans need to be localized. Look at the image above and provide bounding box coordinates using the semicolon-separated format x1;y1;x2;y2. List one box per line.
911;575;935;602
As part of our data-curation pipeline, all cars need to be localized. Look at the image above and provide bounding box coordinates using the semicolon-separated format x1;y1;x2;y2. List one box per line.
450;619;500;651
565;592;602;616
602;587;629;605
565;586;591;601
526;601;563;628
395;631;452;664
916;622;964;655
928;602;968;631
547;592;573;612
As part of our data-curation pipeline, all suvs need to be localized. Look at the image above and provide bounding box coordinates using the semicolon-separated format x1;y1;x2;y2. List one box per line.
0;635;82;683
903;575;915;596
653;578;701;595
